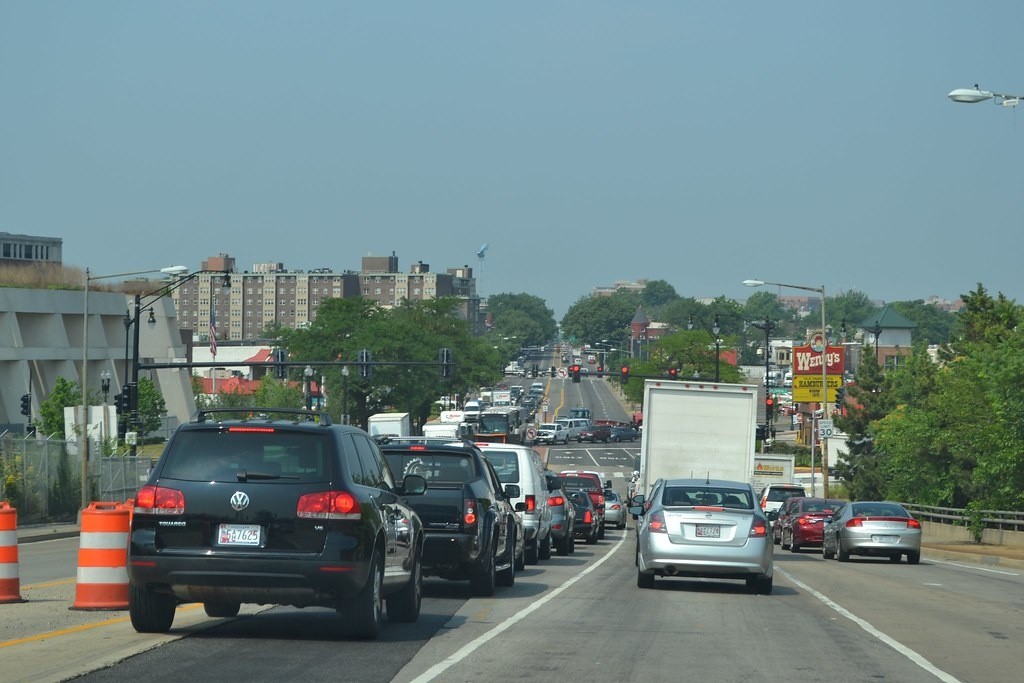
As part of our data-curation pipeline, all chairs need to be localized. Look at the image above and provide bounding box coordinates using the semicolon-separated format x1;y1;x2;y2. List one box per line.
702;494;718;505
720;496;741;505
234;443;264;471
436;457;468;481
298;445;317;475
667;490;693;506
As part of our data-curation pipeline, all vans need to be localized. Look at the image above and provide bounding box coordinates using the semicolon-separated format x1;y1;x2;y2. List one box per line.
437;441;562;565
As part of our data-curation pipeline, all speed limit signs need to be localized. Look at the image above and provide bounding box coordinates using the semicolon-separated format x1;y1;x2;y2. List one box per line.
817;419;834;439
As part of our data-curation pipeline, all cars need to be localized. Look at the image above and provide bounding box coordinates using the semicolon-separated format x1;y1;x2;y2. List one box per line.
817;499;922;564
771;497;836;553
628;478;776;594
368;344;644;555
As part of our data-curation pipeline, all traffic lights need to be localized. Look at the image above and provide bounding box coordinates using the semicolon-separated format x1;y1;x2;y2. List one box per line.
20;394;28;415
597;367;602;378
621;366;629;383
571;364;581;383
568;366;573;378
766;398;773;420
669;369;676;380
114;392;123;414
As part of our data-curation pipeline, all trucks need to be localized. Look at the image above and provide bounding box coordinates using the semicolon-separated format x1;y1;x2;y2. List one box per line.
636;377;760;499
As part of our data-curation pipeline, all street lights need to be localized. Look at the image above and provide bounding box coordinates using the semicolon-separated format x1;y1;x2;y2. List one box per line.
101;369;112;405
128;268;233;456
123;306;157;446
602;339;622;364
303;365;314;422
341;365;350;425
712;314;771;457
741;280;831;501
609;348;634;363
75;264;191;524
595;342;614;356
687;313;728;384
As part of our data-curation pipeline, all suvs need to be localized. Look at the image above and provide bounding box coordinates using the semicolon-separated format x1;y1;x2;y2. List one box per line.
370;435;524;598
755;482;807;532
125;407;430;639
